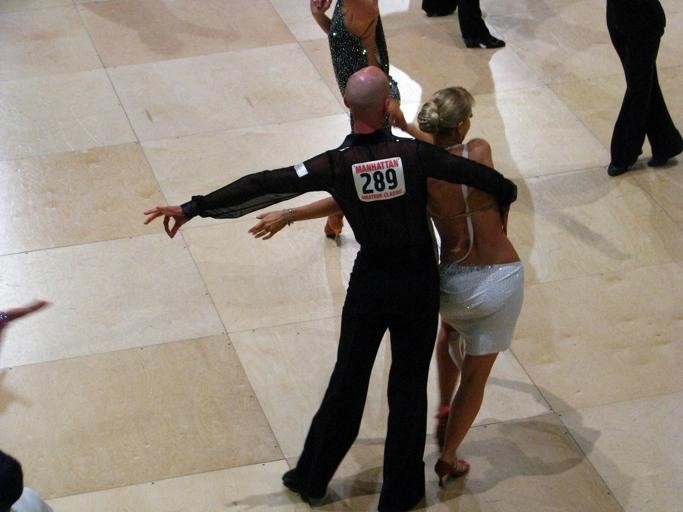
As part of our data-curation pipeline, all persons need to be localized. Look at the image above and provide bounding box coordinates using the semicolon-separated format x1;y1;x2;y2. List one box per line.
141;63;518;512
603;0;681;175
247;83;526;493
308;0;392;244
422;0;506;49
0;299;47;328
0;451;57;512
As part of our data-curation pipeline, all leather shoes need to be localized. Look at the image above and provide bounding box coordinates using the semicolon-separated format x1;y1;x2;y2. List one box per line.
463;32;505;48
608;148;683;176
283;468;321;502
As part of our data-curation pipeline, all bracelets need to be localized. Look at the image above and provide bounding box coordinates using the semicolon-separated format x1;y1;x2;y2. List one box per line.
282;206;296;227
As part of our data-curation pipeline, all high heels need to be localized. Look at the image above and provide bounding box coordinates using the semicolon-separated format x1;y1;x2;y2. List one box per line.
435;410;469;487
324;216;343;241
